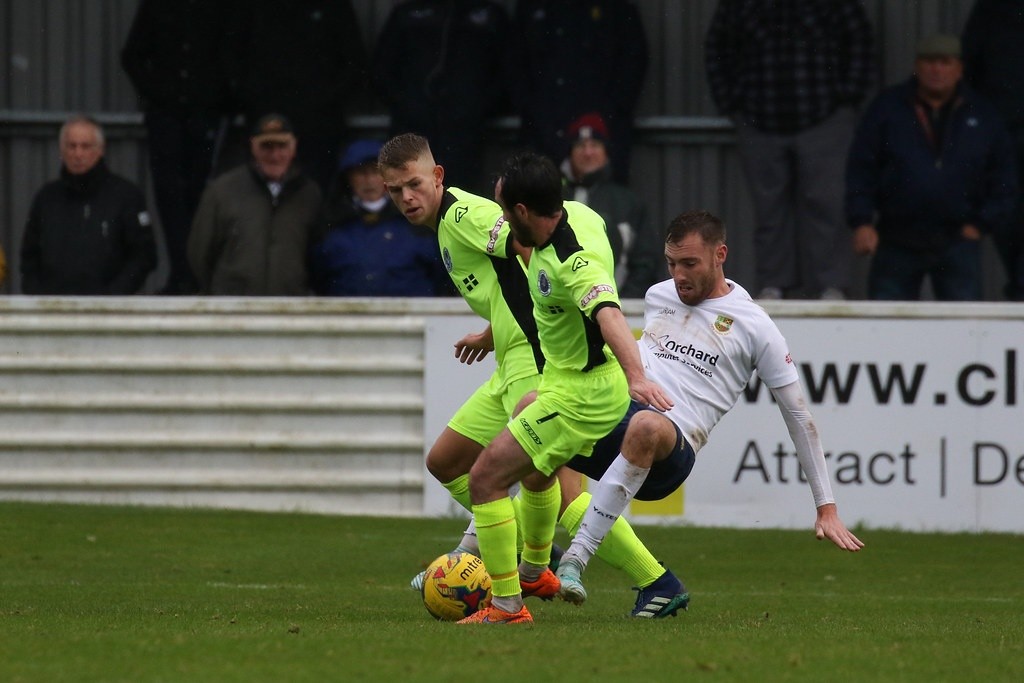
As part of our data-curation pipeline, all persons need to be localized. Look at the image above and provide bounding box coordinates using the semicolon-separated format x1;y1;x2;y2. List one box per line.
14;119;161;297
407;200;867;601
301;136;464;299
374;129;692;620
551;117;669;301
183;110;328;298
846;35;1006;302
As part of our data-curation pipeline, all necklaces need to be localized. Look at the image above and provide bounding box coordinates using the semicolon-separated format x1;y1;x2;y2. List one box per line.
451;152;675;626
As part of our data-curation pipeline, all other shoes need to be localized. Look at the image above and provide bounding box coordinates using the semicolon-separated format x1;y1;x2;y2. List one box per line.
410;544;482;591
548;543;566;575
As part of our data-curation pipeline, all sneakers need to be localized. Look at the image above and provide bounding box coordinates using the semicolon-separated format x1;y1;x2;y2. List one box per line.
455;599;534;625
520;568;561;599
555;559;587;604
630;568;690;618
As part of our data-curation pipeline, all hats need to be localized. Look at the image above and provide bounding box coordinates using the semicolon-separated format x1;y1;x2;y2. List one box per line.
915;32;963;58
251;116;296;142
570;113;608;142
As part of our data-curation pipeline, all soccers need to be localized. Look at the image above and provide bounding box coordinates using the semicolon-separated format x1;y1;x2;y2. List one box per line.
421;551;493;622
